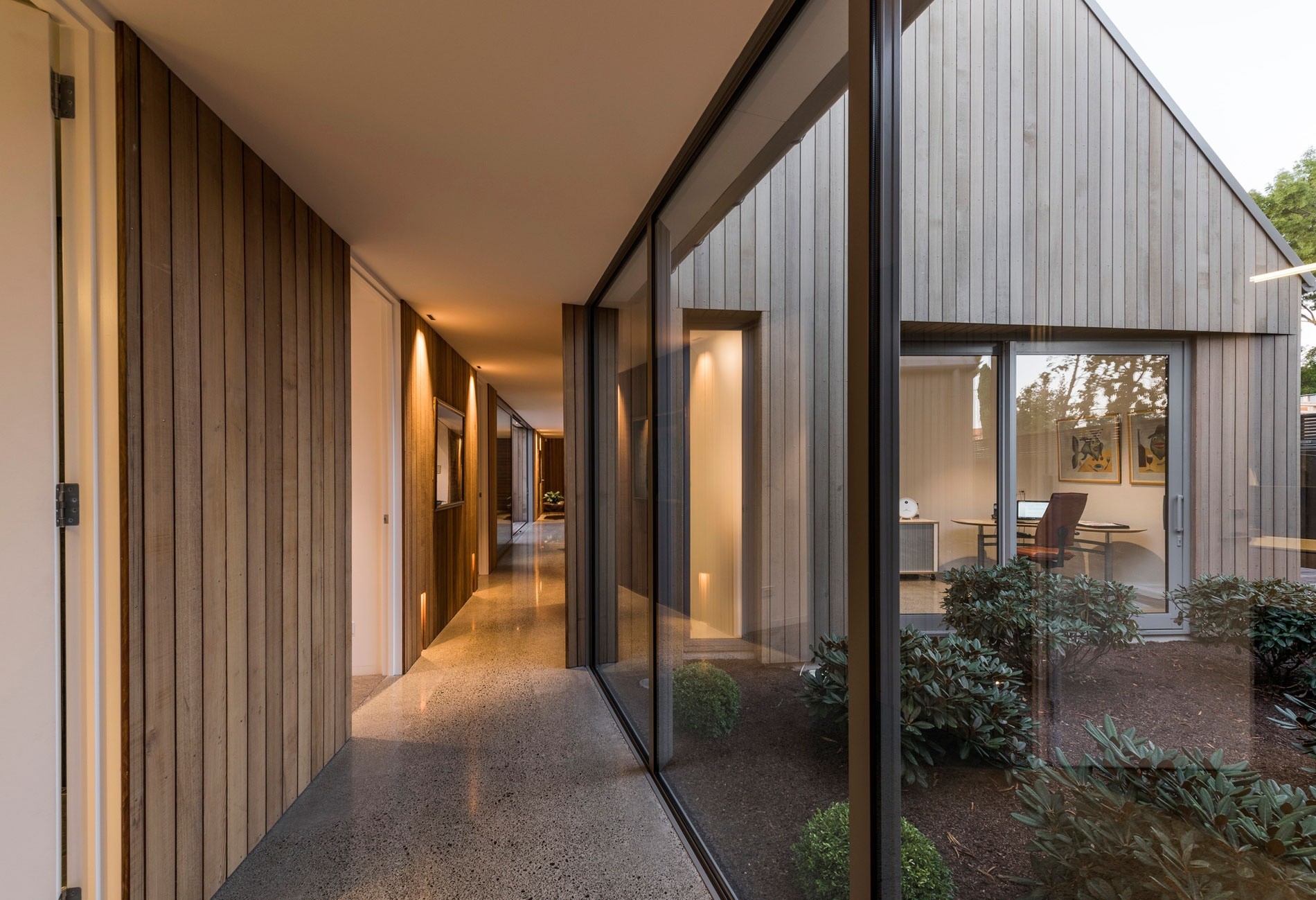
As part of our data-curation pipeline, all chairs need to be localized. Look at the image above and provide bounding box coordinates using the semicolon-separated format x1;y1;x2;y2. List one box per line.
1017;493;1088;573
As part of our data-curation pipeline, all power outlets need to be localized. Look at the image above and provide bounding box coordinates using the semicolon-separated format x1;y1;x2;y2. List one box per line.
1017;488;1026;494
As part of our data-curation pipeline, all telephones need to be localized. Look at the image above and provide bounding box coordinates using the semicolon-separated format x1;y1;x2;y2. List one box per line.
991;503;997;519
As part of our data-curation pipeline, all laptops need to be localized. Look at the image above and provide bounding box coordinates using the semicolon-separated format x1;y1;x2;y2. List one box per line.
1017;500;1050;523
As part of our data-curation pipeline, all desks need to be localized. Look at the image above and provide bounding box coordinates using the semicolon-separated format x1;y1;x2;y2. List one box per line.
952;518;1147;602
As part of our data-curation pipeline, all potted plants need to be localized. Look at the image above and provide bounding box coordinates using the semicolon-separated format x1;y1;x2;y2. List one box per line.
543;491;565;511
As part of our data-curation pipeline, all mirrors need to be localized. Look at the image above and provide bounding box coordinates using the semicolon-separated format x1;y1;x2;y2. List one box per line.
432;400;465;512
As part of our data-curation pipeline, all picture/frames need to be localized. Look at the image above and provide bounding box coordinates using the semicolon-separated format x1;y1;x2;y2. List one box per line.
1057;412;1121;485
1127;408;1166;485
